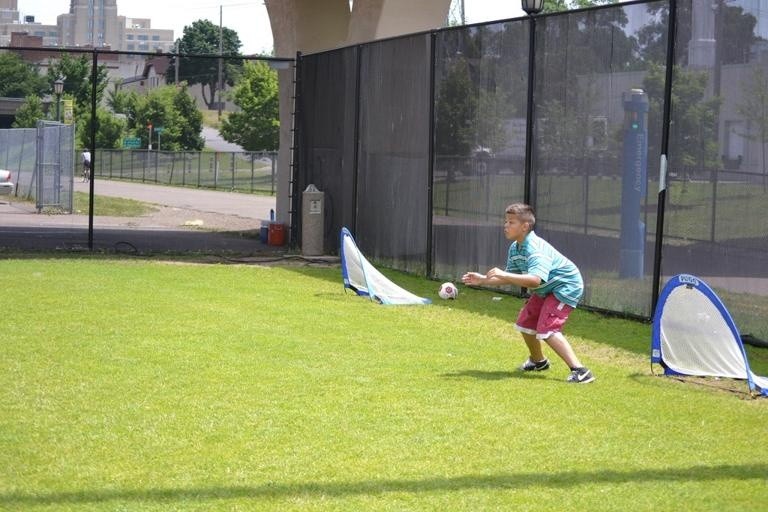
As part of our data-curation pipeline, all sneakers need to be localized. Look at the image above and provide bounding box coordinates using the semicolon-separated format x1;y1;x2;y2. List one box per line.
520;357;551;371
568;367;595;383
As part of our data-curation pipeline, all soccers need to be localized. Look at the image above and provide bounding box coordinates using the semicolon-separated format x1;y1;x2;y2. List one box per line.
438;282;458;300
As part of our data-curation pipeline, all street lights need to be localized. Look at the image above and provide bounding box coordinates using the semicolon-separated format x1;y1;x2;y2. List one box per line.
53;79;64;121
521;0;545;296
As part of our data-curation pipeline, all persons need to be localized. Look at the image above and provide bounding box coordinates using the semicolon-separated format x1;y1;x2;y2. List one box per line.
460;201;596;386
78;147;91;181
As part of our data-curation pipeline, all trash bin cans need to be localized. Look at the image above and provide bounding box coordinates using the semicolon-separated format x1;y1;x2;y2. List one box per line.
300;184;324;256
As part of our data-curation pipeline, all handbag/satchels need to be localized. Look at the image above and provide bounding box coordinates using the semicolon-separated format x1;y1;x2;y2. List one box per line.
85;160;90;166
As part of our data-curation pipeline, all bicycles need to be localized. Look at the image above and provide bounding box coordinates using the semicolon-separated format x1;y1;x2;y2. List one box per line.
80;167;90;183
